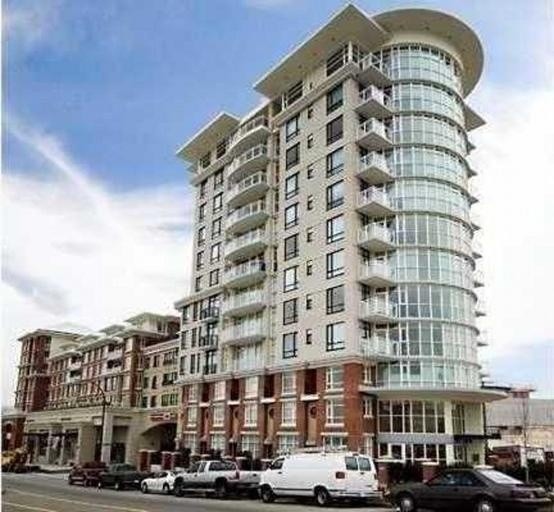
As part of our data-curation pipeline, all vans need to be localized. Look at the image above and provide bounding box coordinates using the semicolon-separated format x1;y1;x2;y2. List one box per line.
257;451;380;506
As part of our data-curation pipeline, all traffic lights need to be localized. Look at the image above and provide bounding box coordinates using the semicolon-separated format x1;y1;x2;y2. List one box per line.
5;424;12;441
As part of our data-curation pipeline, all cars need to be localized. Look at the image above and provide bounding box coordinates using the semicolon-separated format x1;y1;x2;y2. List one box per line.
141;470;185;494
389;465;552;512
98;464;150;491
68;461;110;487
171;460;240;497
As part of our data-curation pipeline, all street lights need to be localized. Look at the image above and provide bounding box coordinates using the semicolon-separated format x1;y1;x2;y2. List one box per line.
72;378;105;461
